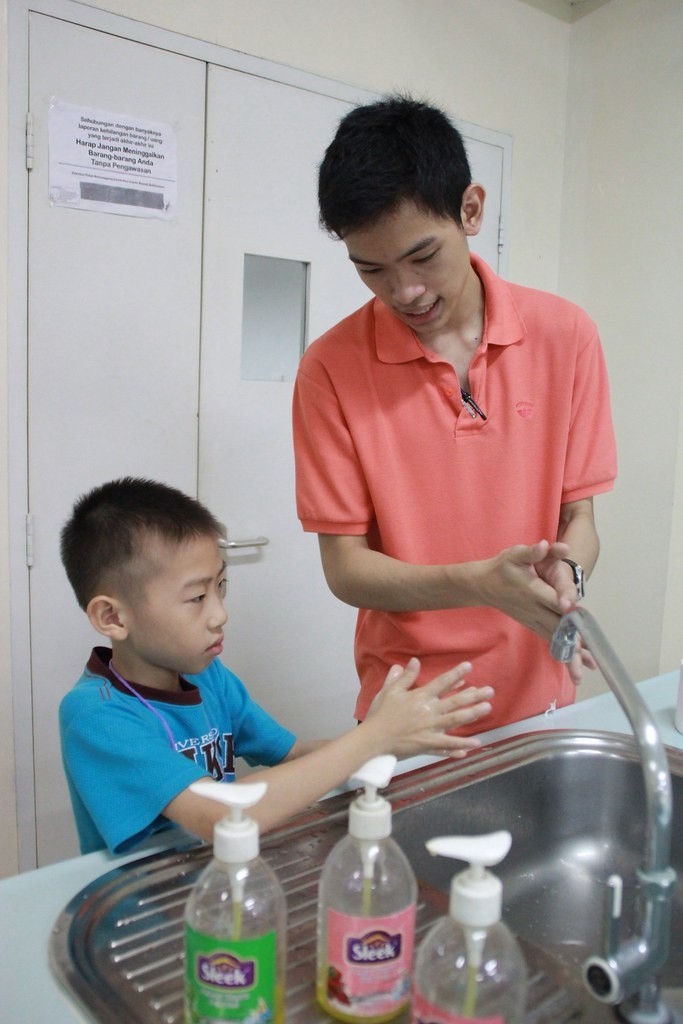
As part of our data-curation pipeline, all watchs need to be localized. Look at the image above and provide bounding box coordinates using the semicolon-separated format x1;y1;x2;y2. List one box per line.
561;557;587;600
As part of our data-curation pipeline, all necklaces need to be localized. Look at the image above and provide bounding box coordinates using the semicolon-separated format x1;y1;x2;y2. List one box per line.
110;658;188;751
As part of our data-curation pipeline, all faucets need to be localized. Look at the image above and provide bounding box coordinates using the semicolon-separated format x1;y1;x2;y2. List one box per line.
548;608;683;1024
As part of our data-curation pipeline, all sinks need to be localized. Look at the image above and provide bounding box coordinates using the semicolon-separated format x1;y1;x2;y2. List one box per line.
298;729;683;1024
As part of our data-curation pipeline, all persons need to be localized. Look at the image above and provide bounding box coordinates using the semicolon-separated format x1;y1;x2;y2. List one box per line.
292;93;617;744
59;478;491;861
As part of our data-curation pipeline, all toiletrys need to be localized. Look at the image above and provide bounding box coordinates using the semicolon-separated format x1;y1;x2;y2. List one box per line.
313;754;418;1024
184;780;288;1024
410;831;531;1024
